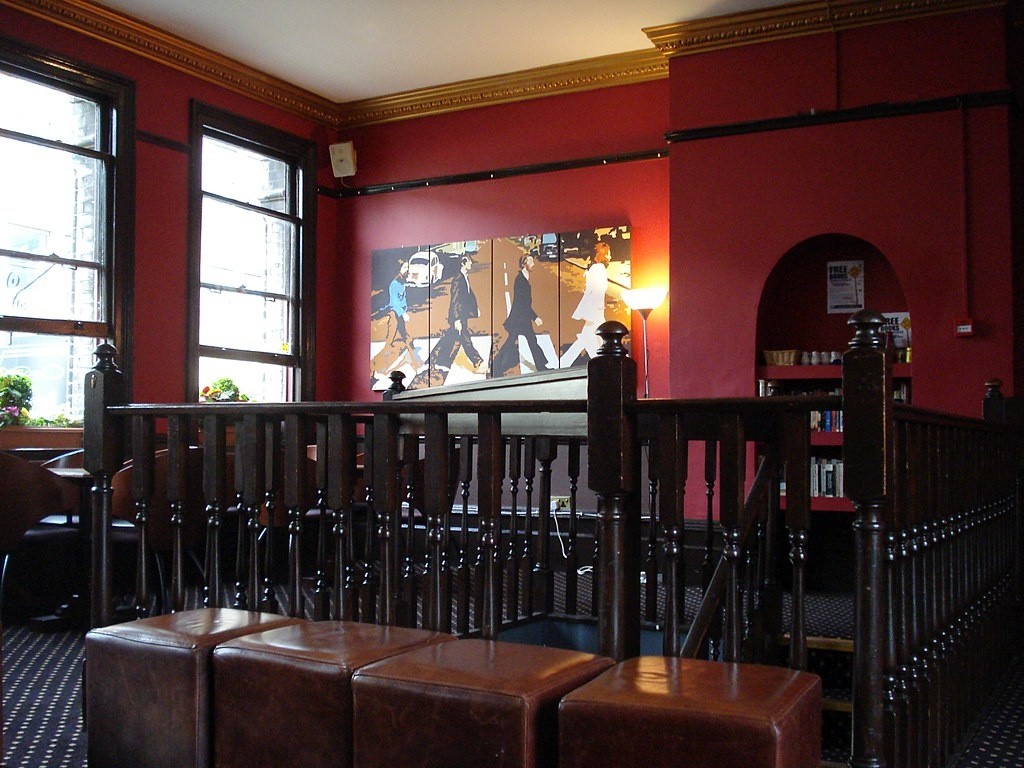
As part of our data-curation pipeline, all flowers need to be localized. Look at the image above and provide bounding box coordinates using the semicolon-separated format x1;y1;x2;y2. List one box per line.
0;404;32;429
199;378;250;402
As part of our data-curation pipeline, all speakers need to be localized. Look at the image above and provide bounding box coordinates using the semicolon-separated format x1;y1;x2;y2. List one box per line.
328;141;356;178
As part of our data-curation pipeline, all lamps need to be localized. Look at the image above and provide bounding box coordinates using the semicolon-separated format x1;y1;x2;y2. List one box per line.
619;286;670;399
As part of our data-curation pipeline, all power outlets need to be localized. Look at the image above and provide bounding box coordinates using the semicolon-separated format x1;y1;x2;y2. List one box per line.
550;495;571;512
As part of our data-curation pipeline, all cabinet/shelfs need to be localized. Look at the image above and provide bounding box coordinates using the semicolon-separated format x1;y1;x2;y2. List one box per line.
753;362;912;514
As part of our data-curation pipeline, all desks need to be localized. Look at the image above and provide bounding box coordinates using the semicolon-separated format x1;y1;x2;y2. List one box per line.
45;467;99;619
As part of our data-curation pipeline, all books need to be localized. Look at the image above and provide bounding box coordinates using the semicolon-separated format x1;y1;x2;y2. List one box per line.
800;384;911;433
775;454;846;499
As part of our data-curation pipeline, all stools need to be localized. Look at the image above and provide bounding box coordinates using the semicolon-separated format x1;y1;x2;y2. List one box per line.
557;655;823;767
350;638;618;768
211;620;459;768
84;607;310;768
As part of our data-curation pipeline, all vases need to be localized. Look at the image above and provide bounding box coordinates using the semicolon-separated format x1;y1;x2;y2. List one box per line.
197;426;236;448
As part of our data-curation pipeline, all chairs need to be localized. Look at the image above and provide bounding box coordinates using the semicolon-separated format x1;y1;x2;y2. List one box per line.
0;444;468;620
